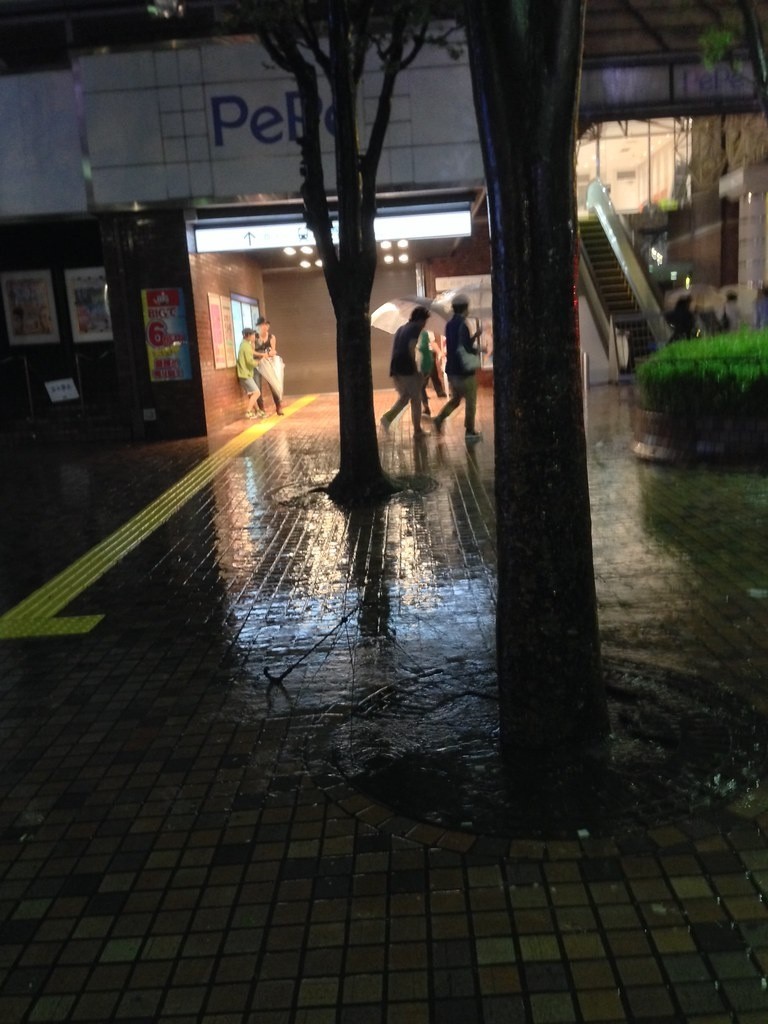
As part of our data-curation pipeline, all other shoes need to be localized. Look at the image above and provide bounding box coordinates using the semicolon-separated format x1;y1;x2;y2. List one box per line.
431;417;442;434
245;410;257;419
465;430;483;438
276;405;284;415
255;409;269;418
421;407;431;415
414;430;432;438
380;416;390;434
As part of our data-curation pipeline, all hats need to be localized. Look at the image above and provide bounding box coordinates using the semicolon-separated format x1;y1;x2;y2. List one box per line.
255;317;271;326
241;328;256;333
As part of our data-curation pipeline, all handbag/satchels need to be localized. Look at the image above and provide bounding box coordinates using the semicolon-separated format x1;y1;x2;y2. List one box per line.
720;307;729;331
459;345;481;372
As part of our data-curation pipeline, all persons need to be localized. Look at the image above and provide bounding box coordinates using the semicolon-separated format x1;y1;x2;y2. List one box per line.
236;328;265;419
379;307;430;439
721;289;745;332
664;294;697;348
252;316;285;417
425;330;451;398
428;295;488;440
752;282;768;327
415;330;437;414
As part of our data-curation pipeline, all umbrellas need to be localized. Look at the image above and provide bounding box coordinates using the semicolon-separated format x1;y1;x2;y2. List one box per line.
370;296;452;339
664;284;722;313
432;279;493;314
255;347;285;400
718;282;763;310
